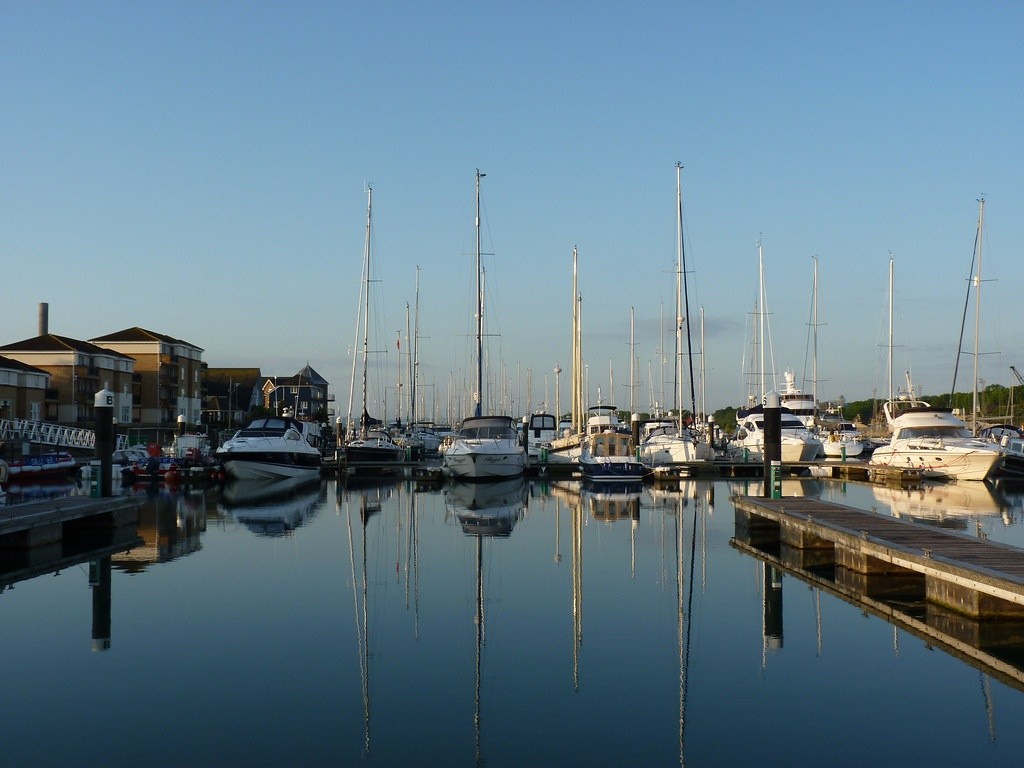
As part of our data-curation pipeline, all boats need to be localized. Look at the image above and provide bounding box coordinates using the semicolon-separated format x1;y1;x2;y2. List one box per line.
211;415;324;481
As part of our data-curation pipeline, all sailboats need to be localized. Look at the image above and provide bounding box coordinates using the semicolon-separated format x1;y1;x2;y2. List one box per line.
327;162;1023;488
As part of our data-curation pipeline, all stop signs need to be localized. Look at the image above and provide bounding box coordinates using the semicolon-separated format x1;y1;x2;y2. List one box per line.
185;448;194;457
